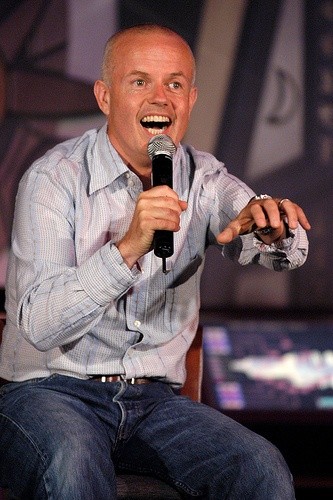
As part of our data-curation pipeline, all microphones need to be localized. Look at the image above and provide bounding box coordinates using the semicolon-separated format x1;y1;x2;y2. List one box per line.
148;133;175;274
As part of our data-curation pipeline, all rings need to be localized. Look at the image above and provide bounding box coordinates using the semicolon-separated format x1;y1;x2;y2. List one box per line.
279;198;292;210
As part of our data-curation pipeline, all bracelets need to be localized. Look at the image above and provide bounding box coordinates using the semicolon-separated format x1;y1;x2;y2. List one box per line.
245;194;276;234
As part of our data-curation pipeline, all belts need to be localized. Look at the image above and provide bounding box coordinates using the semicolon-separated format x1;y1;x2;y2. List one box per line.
91;374;154;384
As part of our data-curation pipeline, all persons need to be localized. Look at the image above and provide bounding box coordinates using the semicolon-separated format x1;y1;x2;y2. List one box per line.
1;25;312;500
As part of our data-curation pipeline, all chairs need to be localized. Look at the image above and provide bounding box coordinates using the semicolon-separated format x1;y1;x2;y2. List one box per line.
113;323;206;499
